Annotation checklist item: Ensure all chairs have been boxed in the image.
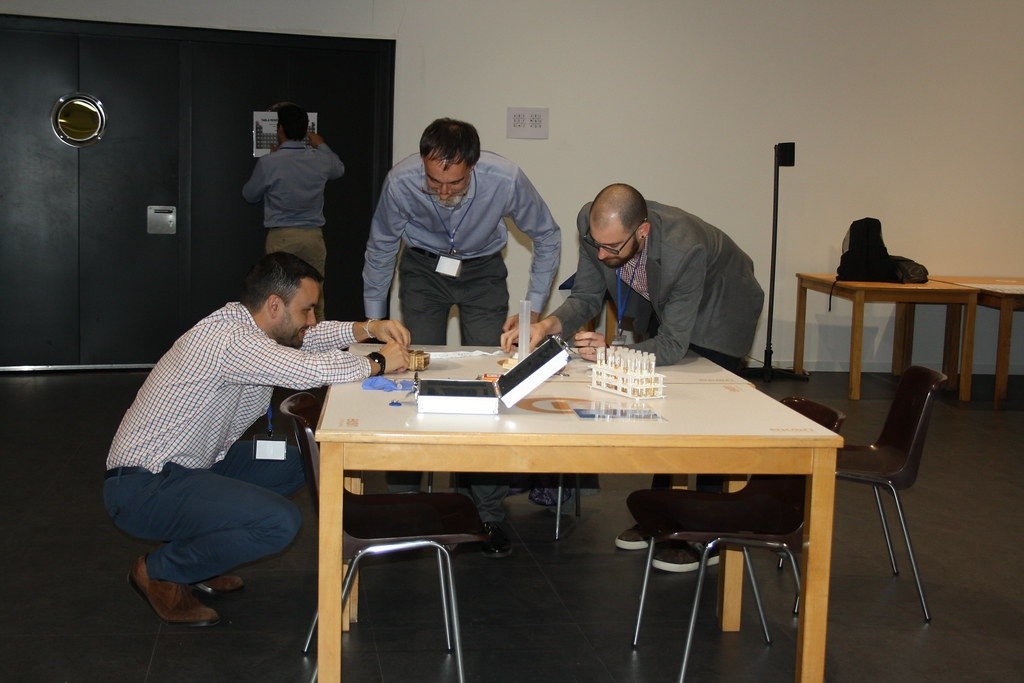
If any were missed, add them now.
[625,397,847,683]
[776,364,948,623]
[279,391,490,683]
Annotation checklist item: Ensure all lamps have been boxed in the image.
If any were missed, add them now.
[752,142,795,383]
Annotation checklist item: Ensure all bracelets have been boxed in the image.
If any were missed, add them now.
[364,319,378,338]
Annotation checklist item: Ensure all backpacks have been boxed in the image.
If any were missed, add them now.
[837,218,928,284]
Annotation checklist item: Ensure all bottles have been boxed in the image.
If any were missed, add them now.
[596,344,657,397]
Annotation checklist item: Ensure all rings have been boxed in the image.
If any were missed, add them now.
[592,347,596,354]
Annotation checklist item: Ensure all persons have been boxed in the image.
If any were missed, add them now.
[244,106,345,324]
[502,184,765,573]
[104,252,411,625]
[362,117,562,559]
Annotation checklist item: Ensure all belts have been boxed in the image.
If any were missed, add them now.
[103,467,150,479]
[409,246,483,263]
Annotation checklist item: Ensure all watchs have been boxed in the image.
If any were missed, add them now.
[367,352,385,376]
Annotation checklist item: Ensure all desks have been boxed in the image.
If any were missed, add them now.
[313,346,844,683]
[902,277,1024,411]
[793,272,982,401]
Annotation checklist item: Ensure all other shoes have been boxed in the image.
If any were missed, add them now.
[128,553,220,628]
[480,520,513,558]
[614,521,659,550]
[187,575,246,595]
[651,538,720,573]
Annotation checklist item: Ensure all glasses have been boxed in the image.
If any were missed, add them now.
[582,217,648,255]
[420,158,472,196]
